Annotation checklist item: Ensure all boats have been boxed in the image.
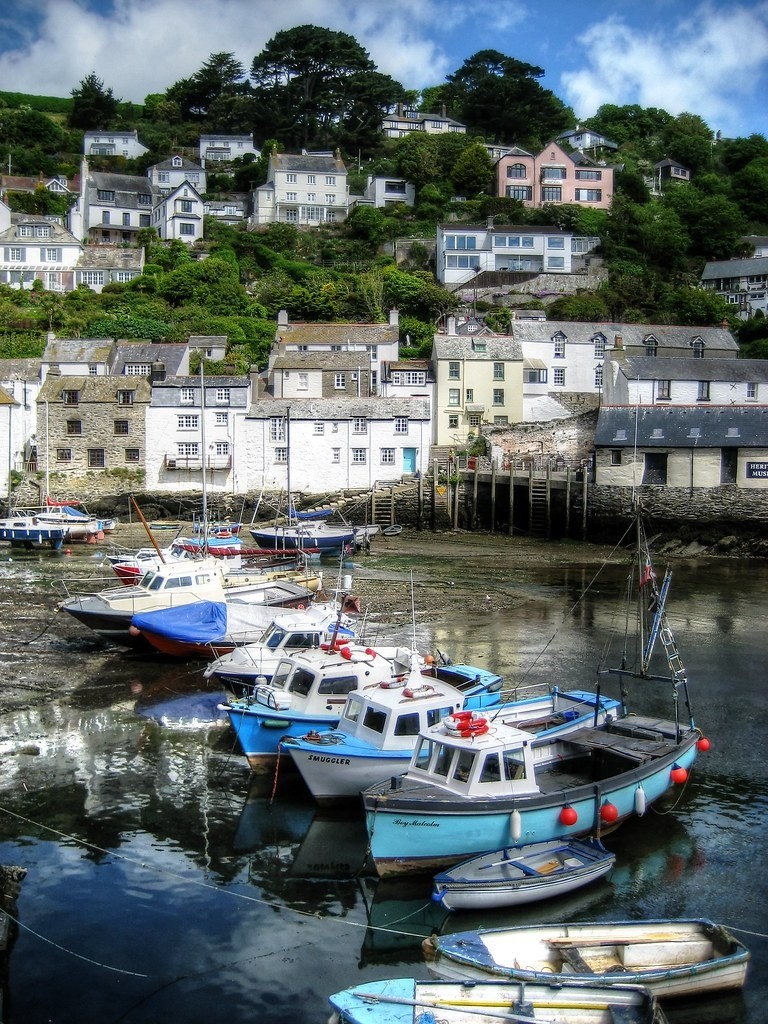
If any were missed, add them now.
[214,591,505,779]
[279,800,377,886]
[326,977,670,1024]
[357,799,700,969]
[438,876,617,943]
[430,833,616,926]
[228,769,317,856]
[359,373,708,885]
[420,916,754,1007]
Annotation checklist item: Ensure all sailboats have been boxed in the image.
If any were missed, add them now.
[280,566,621,812]
[0,395,460,735]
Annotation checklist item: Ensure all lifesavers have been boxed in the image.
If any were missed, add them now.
[403,684,434,698]
[379,677,409,689]
[216,531,230,538]
[342,645,377,662]
[321,638,355,650]
[445,710,489,737]
